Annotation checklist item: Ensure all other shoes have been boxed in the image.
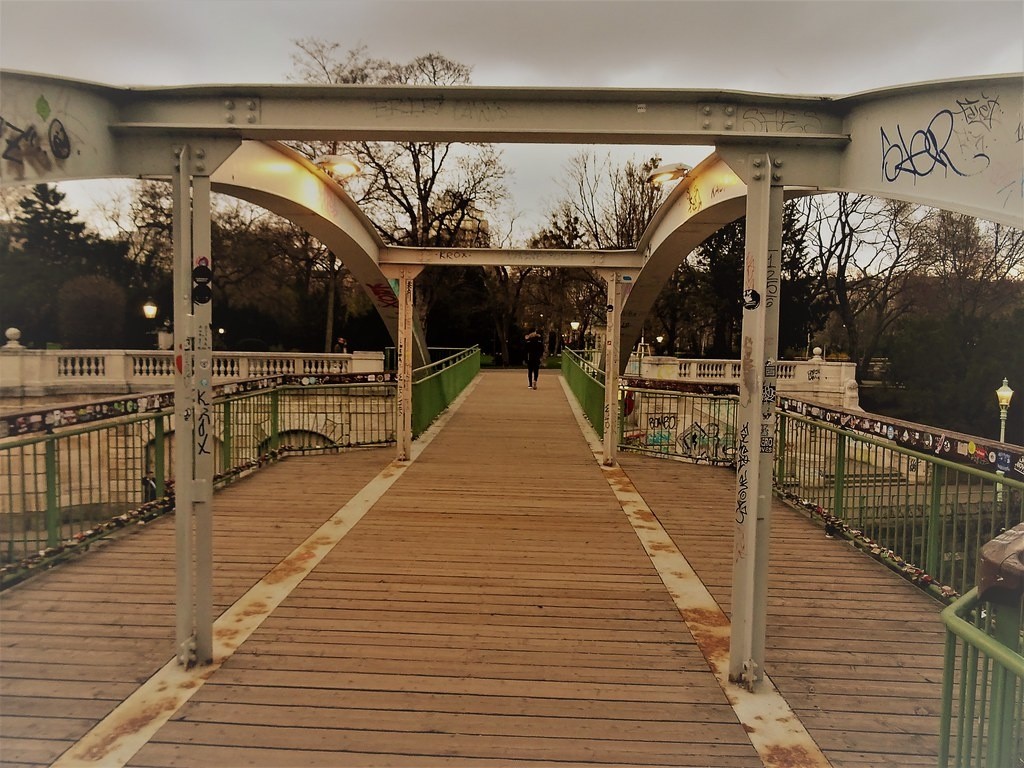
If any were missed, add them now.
[528,386,532,388]
[533,381,537,390]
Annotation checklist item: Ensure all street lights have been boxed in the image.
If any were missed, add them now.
[994,377,1013,513]
[570,317,580,352]
[312,155,361,352]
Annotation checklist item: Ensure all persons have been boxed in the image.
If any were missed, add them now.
[523,322,544,390]
[334,338,351,369]
